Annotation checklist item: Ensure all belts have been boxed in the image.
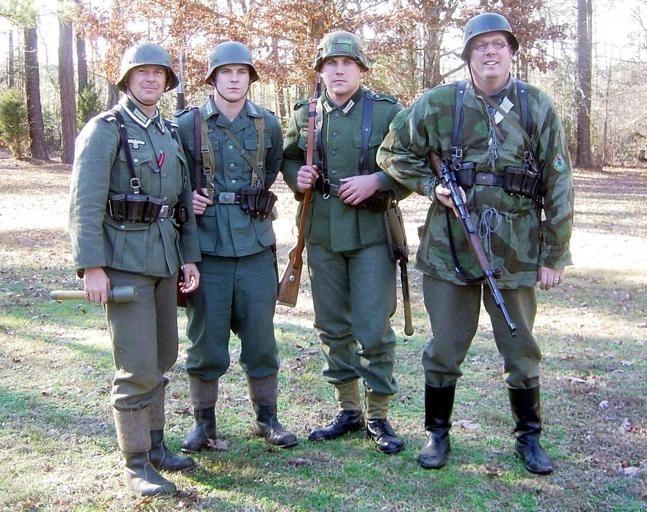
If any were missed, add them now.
[214,193,241,205]
[158,205,175,218]
[474,173,504,187]
[322,182,341,196]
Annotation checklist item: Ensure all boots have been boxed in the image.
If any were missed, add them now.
[418,382,455,469]
[508,384,554,475]
[367,389,404,454]
[308,379,365,440]
[113,394,196,496]
[246,374,299,448]
[181,375,218,453]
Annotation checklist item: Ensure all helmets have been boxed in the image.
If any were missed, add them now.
[461,13,519,60]
[204,42,259,85]
[115,42,179,92]
[313,32,369,72]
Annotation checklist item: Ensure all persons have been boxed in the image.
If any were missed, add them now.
[70,42,202,497]
[279,30,413,454]
[176,41,299,454]
[376,13,574,475]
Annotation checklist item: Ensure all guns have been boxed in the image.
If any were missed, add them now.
[429,151,518,337]
[274,71,321,307]
[173,55,187,307]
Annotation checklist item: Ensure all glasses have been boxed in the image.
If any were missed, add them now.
[473,39,505,51]
